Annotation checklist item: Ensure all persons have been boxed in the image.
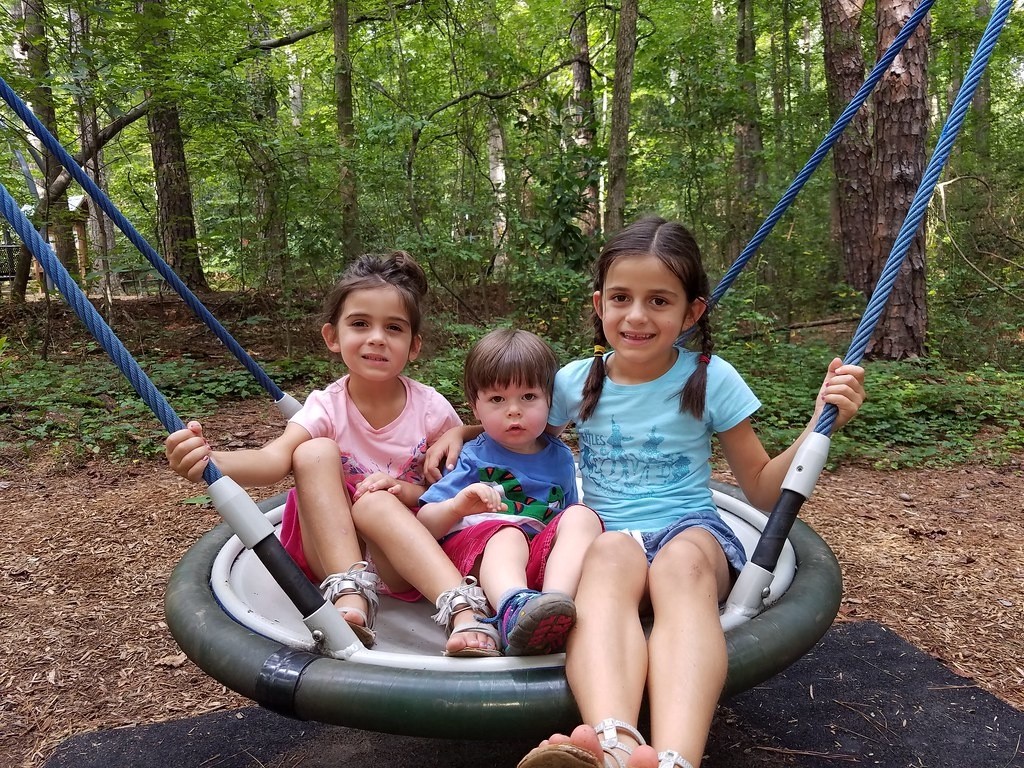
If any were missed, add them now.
[417,327,605,657]
[163,248,465,652]
[419,217,868,767]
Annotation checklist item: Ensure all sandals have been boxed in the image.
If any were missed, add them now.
[517,718,647,768]
[430,575,503,658]
[318,562,380,646]
[657,749,696,768]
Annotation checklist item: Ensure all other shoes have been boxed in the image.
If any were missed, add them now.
[473,588,577,657]
